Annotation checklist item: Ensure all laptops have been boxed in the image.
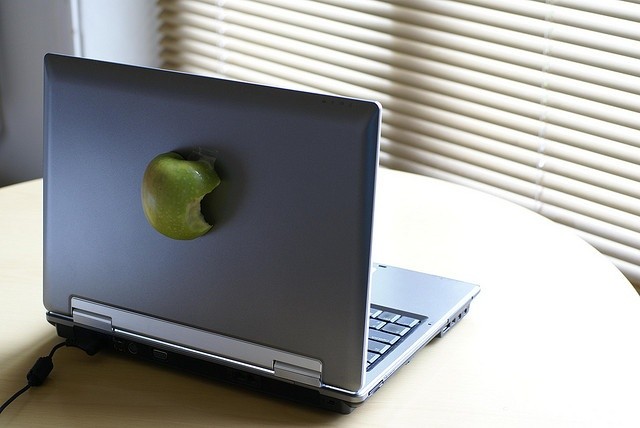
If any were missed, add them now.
[43,51,480,415]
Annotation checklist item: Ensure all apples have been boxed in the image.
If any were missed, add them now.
[141,152,221,240]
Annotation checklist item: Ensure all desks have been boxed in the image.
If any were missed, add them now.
[0,163,636,427]
